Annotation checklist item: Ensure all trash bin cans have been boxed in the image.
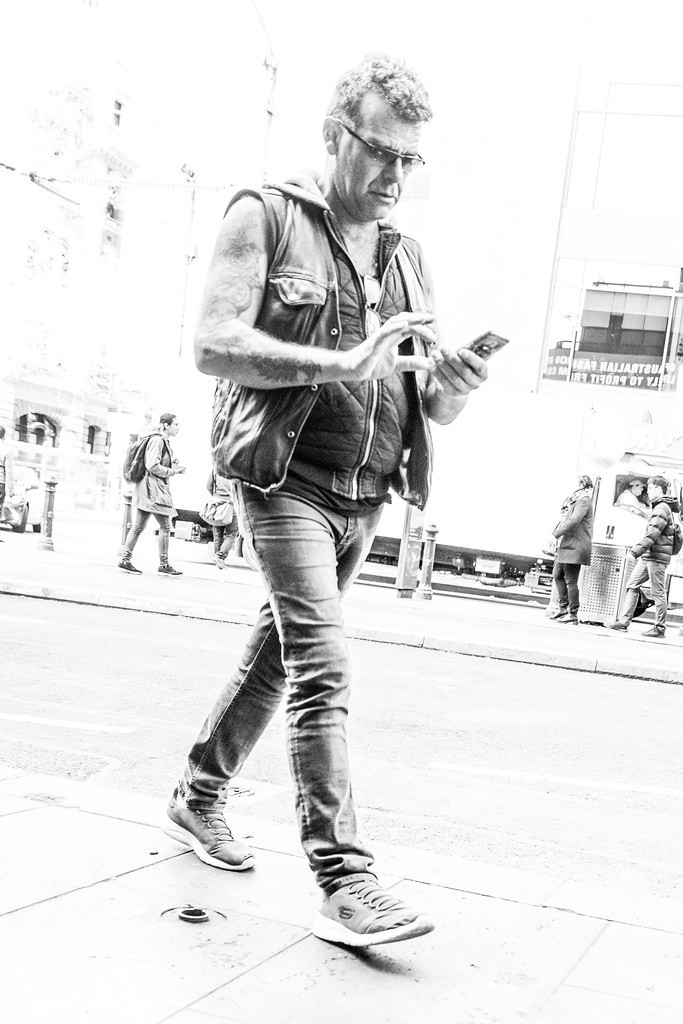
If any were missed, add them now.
[544,541,642,626]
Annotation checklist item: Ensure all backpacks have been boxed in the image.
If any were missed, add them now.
[123,437,150,484]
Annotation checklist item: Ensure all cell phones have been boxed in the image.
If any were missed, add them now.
[469,331,512,361]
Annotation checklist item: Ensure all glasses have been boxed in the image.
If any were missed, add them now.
[335,119,426,173]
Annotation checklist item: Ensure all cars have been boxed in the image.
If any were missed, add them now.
[1,461,50,534]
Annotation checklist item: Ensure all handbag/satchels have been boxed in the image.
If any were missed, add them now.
[672,524,683,555]
[541,520,561,558]
[199,497,233,526]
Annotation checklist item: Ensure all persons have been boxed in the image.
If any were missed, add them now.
[204,463,240,571]
[157,54,491,948]
[549,475,594,625]
[615,479,647,515]
[603,475,675,638]
[116,414,185,578]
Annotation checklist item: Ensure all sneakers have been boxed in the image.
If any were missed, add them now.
[313,880,435,946]
[161,786,256,871]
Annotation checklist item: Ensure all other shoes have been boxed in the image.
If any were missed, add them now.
[212,555,227,570]
[641,626,664,638]
[557,611,579,625]
[118,560,142,575]
[550,609,569,620]
[158,565,183,577]
[606,622,628,632]
